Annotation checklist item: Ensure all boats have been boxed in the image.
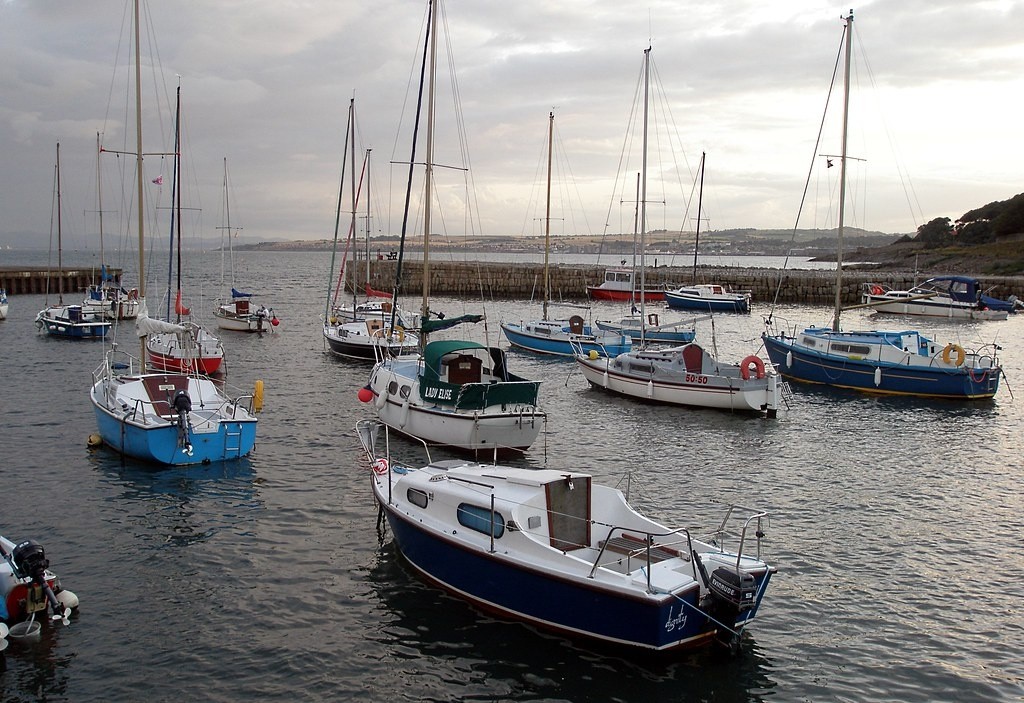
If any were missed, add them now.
[353,414,780,665]
[862,272,1024,322]
[0,292,11,320]
[1,536,79,643]
[585,267,679,300]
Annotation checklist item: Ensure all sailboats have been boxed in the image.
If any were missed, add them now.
[563,37,786,419]
[501,106,634,356]
[82,133,141,319]
[35,139,112,340]
[212,155,278,334]
[142,85,227,375]
[595,172,698,344]
[757,8,1006,406]
[92,0,259,468]
[323,90,423,360]
[665,148,752,315]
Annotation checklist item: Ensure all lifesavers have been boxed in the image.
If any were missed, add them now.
[388,326,404,342]
[253,380,263,413]
[870,286,883,295]
[943,344,966,366]
[741,355,764,379]
[128,288,138,299]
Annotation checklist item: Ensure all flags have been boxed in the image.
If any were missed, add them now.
[152,175,163,185]
